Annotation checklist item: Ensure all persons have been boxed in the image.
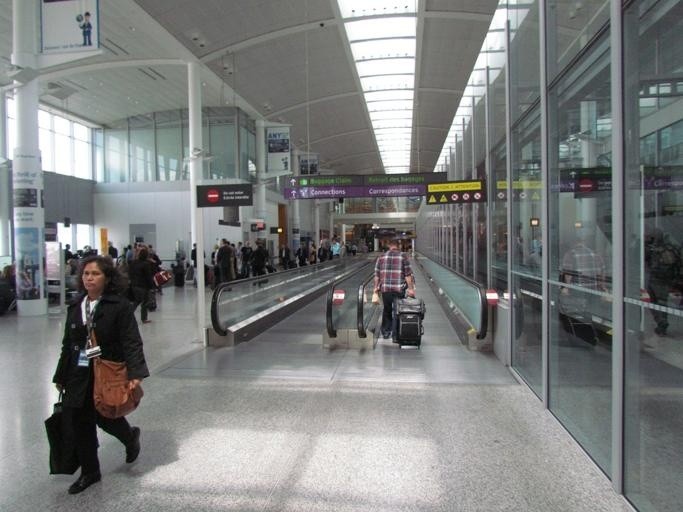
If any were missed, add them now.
[50,254,150,494]
[169,233,416,291]
[371,238,415,344]
[476,218,543,286]
[78,10,93,46]
[0,232,164,326]
[557,219,680,334]
[282,157,288,170]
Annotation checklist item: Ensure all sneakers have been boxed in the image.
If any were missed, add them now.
[142,320,152,324]
[384,330,399,343]
[654,322,668,334]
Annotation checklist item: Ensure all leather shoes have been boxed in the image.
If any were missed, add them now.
[126,427,141,463]
[69,473,100,494]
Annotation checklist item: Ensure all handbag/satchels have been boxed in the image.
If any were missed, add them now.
[153,270,172,287]
[44,388,100,476]
[93,356,144,419]
[396,298,425,319]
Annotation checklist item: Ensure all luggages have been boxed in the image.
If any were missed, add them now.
[397,313,422,350]
